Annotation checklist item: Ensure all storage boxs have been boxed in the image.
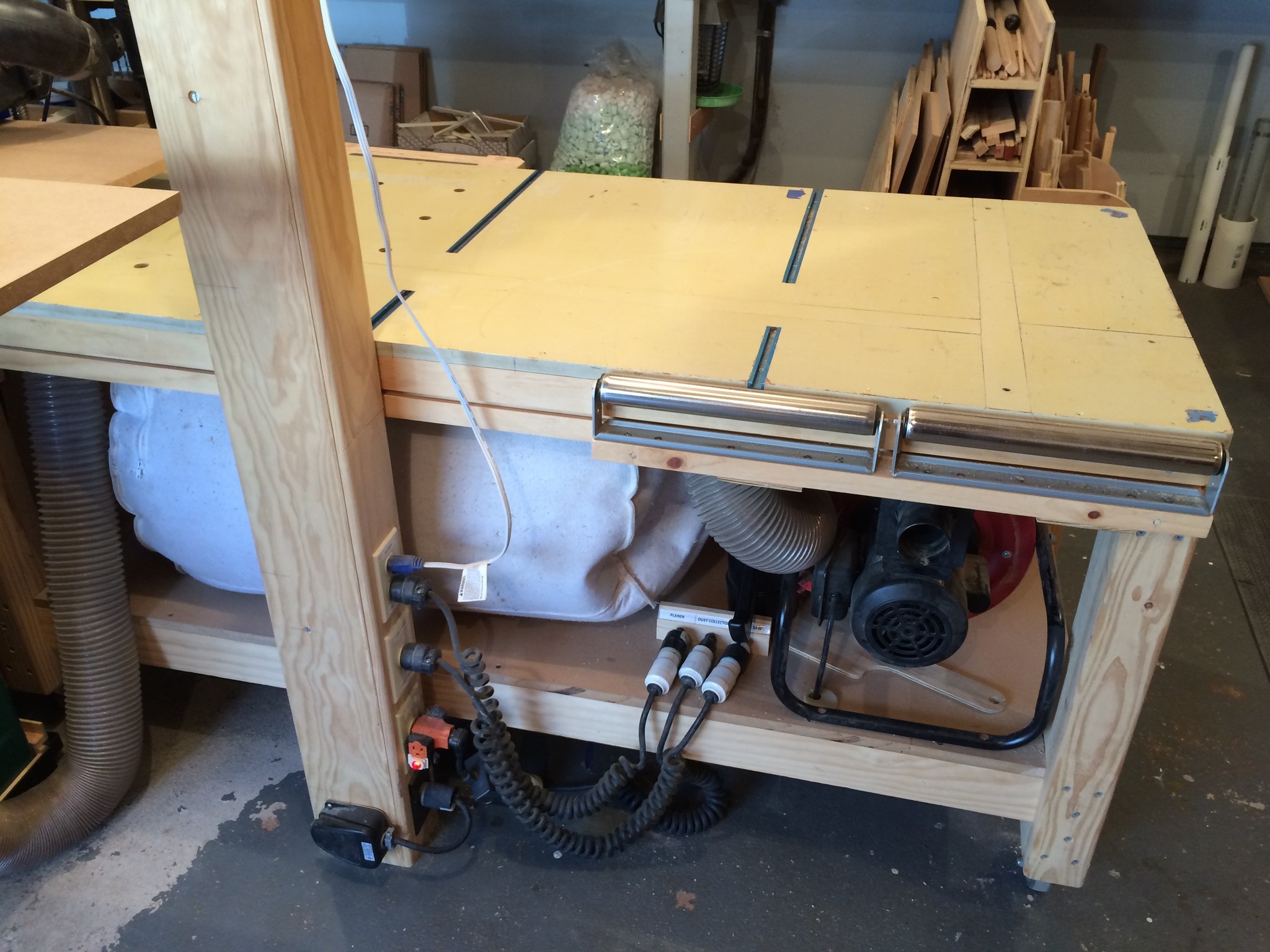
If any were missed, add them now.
[336,43,537,168]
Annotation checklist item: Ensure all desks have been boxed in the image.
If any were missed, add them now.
[0,179,182,313]
[0,122,167,184]
[0,122,1236,888]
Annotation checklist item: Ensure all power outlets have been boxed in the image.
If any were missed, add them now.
[372,527,402,623]
[396,681,420,778]
[384,606,417,704]
